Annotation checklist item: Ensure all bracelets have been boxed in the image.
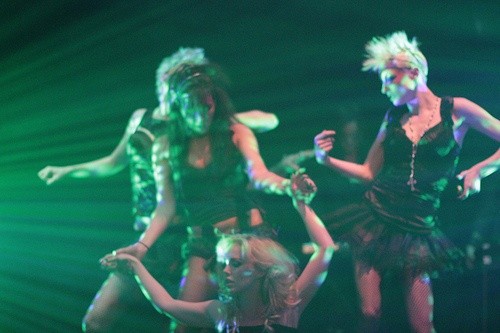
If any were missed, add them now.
[136,239,151,252]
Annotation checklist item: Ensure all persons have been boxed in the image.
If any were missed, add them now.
[38,48,336,333]
[313,31,500,333]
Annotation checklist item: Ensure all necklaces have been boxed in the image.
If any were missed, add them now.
[405,97,438,190]
